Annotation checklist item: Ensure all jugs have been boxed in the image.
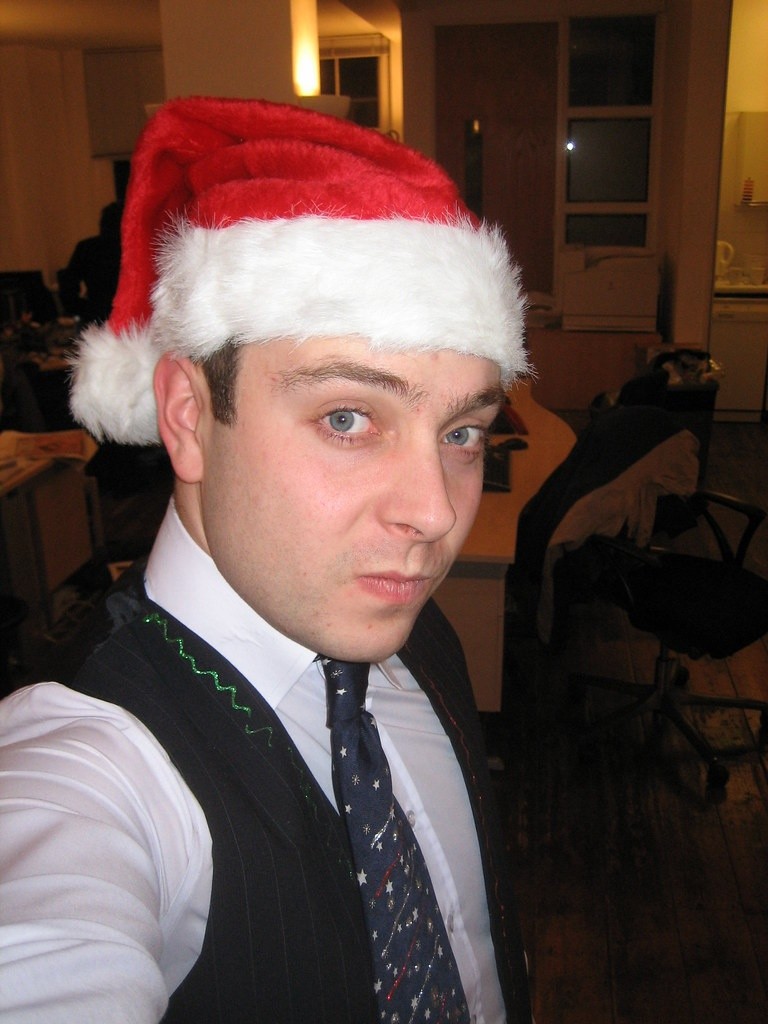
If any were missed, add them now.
[714,239,734,276]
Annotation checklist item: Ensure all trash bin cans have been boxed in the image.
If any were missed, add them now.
[431,372,578,710]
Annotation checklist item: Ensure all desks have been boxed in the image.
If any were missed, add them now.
[432,371,576,711]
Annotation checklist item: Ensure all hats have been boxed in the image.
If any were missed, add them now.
[68,97,529,445]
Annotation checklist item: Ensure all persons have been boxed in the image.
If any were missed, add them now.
[59,202,126,325]
[0,94,539,1024]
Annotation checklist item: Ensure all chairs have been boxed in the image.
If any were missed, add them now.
[562,409,768,789]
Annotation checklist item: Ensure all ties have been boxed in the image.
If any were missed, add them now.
[317,650,472,1024]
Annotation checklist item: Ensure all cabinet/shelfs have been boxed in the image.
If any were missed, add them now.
[554,13,668,333]
[709,297,768,421]
[0,429,106,638]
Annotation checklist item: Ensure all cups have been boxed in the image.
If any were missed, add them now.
[750,268,767,285]
[725,267,741,284]
